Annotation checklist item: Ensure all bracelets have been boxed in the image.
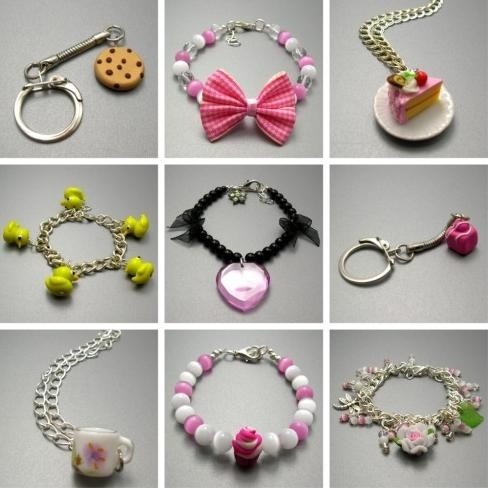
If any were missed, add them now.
[172,16,319,147]
[165,178,321,315]
[171,343,317,471]
[334,353,486,462]
[1,185,155,298]
